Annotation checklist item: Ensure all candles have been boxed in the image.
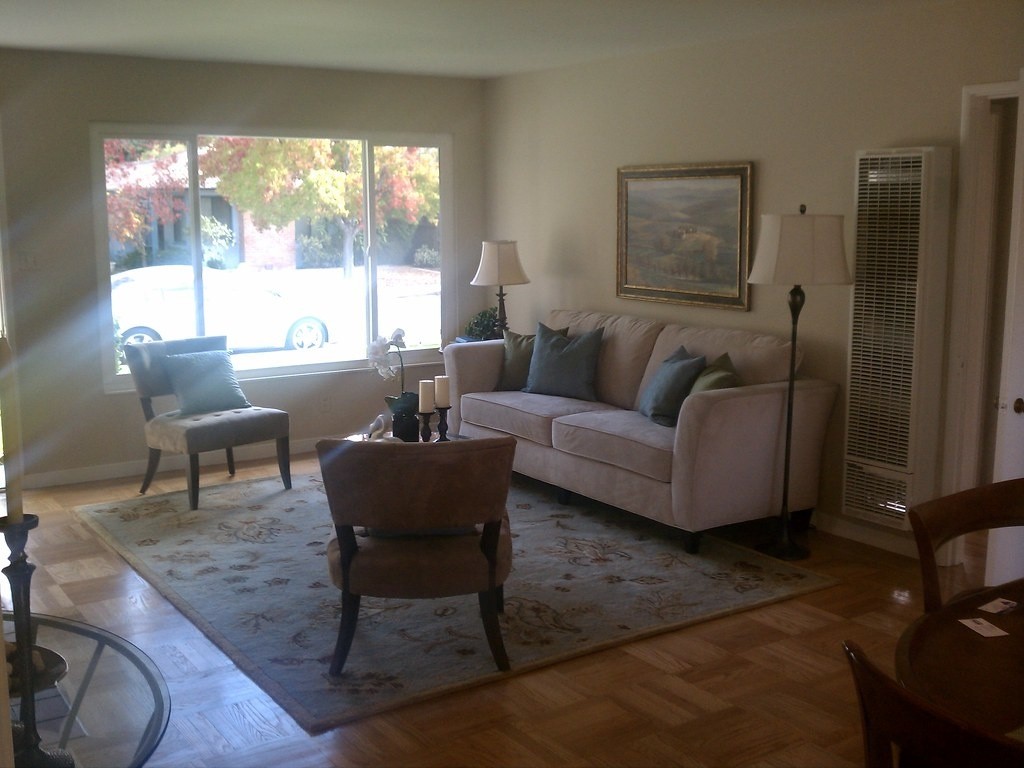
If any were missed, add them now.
[433,376,450,408]
[418,380,434,413]
[0,329,24,524]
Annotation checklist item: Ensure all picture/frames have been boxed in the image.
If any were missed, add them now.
[615,162,750,312]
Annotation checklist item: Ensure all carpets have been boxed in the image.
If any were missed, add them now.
[69,469,840,733]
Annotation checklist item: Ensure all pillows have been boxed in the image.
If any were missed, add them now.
[639,345,707,426]
[492,327,569,391]
[520,321,604,401]
[689,352,737,393]
[162,349,250,415]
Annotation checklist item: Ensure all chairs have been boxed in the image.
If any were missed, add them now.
[907,478,1024,612]
[121,336,291,510]
[315,436,516,673]
[841,639,1024,768]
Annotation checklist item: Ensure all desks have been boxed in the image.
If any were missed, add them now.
[894,590,1024,741]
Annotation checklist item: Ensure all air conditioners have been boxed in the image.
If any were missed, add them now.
[841,145,952,532]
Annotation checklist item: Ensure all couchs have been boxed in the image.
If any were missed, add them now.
[442,309,840,553]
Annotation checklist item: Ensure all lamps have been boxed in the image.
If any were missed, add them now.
[469,240,530,341]
[745,204,853,562]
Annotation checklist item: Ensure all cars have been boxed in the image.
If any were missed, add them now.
[111,264,337,365]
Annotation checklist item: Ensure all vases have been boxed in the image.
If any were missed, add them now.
[391,414,419,442]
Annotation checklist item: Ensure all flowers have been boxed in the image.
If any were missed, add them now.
[367,328,419,419]
